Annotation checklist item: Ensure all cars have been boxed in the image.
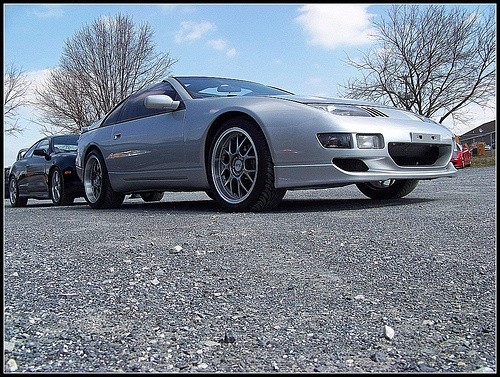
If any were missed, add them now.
[74,74,459,212]
[449,142,472,169]
[8,134,164,206]
